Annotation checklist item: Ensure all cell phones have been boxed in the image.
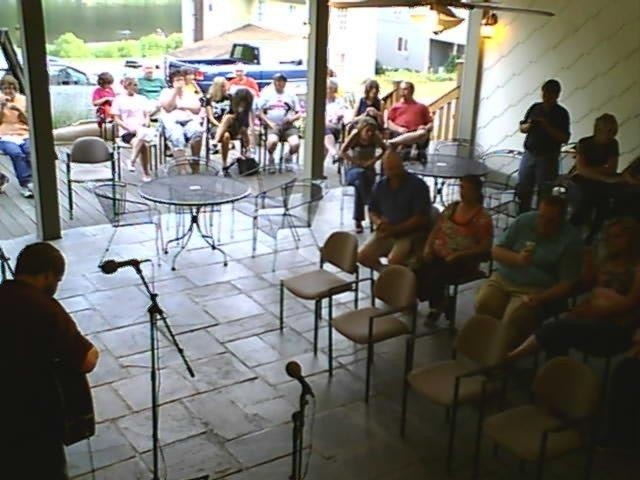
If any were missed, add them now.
[527,242,536,247]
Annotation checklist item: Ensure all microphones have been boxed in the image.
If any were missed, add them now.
[285,360,317,399]
[97,259,139,274]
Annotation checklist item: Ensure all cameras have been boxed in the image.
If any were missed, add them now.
[200,97,210,107]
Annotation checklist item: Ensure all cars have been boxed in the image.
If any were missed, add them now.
[45,55,116,126]
[120,55,165,90]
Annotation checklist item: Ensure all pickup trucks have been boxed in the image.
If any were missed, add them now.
[165,42,333,94]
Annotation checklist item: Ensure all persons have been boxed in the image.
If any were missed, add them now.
[518,77,570,210]
[508,218,638,364]
[93,64,300,175]
[336,115,386,234]
[324,68,433,177]
[0,243,99,480]
[1,73,36,198]
[569,112,637,204]
[473,195,598,334]
[357,150,431,272]
[416,173,494,325]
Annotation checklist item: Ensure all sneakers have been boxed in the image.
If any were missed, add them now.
[21,186,33,198]
[127,153,294,183]
[427,296,455,321]
[355,220,363,233]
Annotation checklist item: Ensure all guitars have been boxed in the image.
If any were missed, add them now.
[0,249,94,445]
